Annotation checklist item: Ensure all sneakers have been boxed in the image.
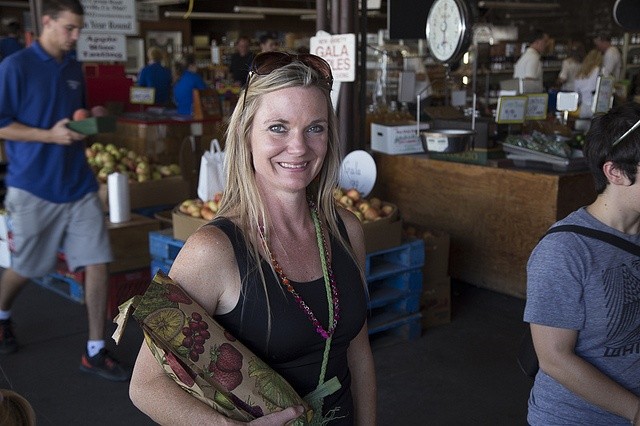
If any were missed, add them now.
[79,347,132,382]
[0,317,18,355]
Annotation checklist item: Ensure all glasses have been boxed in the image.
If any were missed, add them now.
[243,51,333,109]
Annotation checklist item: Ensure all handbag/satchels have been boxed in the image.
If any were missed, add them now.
[197,138,229,202]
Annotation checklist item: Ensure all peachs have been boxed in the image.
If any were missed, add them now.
[72,109,89,121]
[91,106,109,118]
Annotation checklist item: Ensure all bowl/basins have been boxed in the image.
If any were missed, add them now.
[419,128,474,153]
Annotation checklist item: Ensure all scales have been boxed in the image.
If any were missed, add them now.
[420,0,478,154]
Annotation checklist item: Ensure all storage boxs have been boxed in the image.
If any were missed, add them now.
[356,204,404,252]
[170,204,210,239]
[420,280,450,327]
[86,143,189,209]
[409,225,448,282]
[370,119,430,154]
[106,214,162,275]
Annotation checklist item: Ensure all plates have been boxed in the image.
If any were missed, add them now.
[339,151,376,198]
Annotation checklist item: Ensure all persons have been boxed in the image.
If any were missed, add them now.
[572,47,609,120]
[128,50,379,426]
[137,45,172,104]
[171,57,209,115]
[0,21,22,59]
[510,29,549,92]
[258,33,279,52]
[592,31,622,81]
[523,100,639,426]
[0,1,132,383]
[227,37,255,83]
[554,39,585,90]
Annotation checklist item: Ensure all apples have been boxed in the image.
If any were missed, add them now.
[406,225,432,242]
[333,189,392,223]
[177,194,225,219]
[86,143,180,180]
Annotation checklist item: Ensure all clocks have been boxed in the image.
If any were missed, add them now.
[425,0,472,69]
